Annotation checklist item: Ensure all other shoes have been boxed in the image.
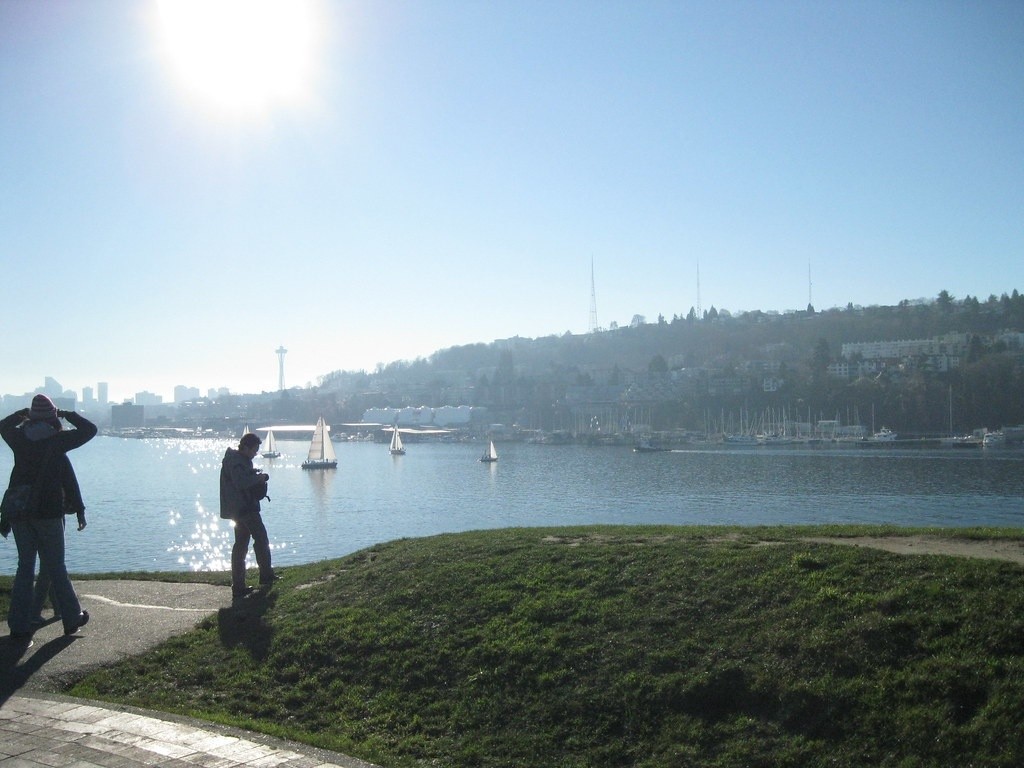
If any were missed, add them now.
[65,611,89,634]
[233,585,253,597]
[54,612,62,618]
[260,576,283,584]
[10,630,24,637]
[32,615,45,624]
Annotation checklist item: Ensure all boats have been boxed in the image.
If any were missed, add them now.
[917,383,1009,447]
[631,433,672,453]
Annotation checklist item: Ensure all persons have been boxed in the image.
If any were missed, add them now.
[220,433,282,597]
[0,394,98,638]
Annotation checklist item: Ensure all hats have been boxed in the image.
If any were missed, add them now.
[30,394,57,420]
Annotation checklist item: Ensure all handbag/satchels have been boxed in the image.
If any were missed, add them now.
[252,481,267,500]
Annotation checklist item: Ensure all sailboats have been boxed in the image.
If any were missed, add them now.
[259,429,281,458]
[301,415,339,468]
[698,401,898,445]
[389,423,408,456]
[478,441,499,462]
